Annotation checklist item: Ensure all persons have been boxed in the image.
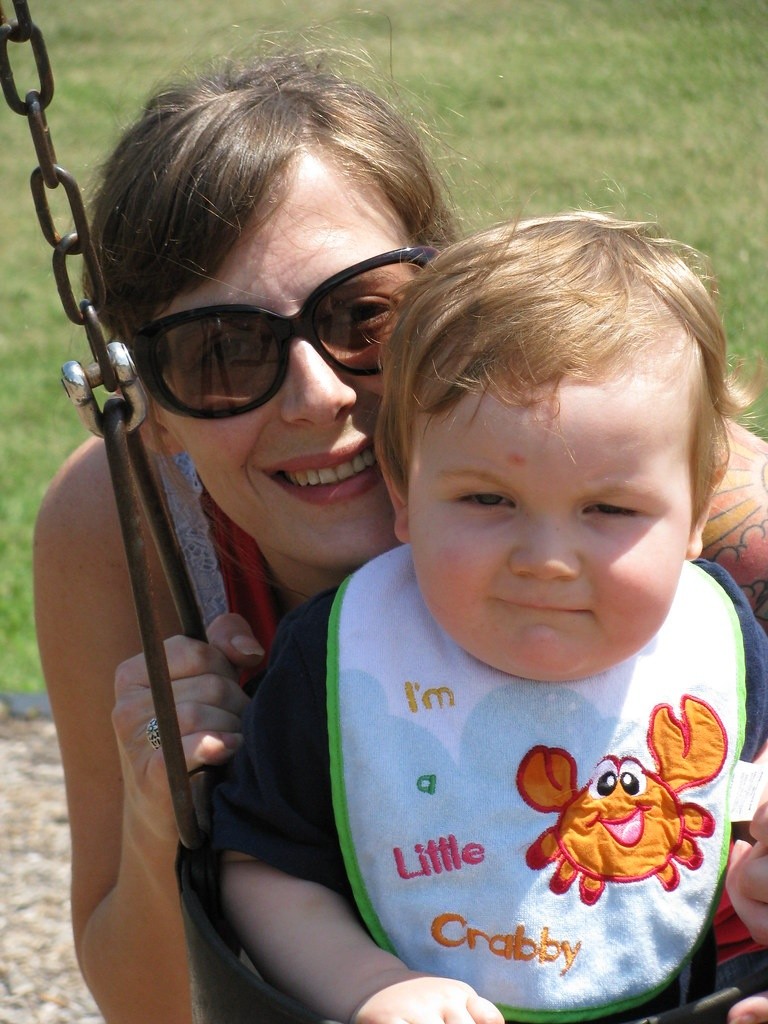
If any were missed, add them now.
[205,210,767,1024]
[31,68,768,1024]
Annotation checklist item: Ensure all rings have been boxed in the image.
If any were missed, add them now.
[146,719,161,750]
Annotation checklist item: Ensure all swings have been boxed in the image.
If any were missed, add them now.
[0,0,768,1024]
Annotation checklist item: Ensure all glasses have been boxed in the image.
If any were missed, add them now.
[129,246,441,420]
